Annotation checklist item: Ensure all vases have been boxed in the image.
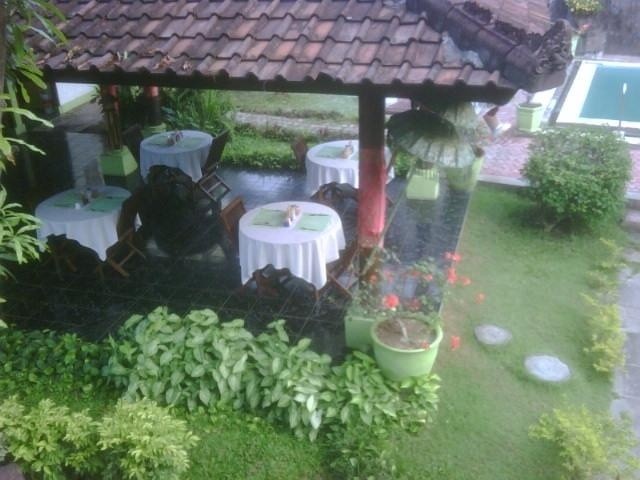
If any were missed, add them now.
[345,306,395,352]
[516,100,543,135]
[445,142,486,193]
[371,313,443,382]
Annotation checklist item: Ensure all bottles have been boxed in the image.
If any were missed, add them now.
[168,129,184,144]
[75,188,92,209]
[283,202,301,228]
[342,141,355,158]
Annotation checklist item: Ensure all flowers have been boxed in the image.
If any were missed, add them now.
[348,223,399,317]
[361,247,487,353]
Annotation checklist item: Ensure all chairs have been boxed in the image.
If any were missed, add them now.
[290,136,327,202]
[350,143,400,210]
[310,234,361,302]
[221,198,281,299]
[19,121,234,283]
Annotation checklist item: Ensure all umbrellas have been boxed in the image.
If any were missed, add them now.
[385,109,475,170]
[412,97,479,129]
